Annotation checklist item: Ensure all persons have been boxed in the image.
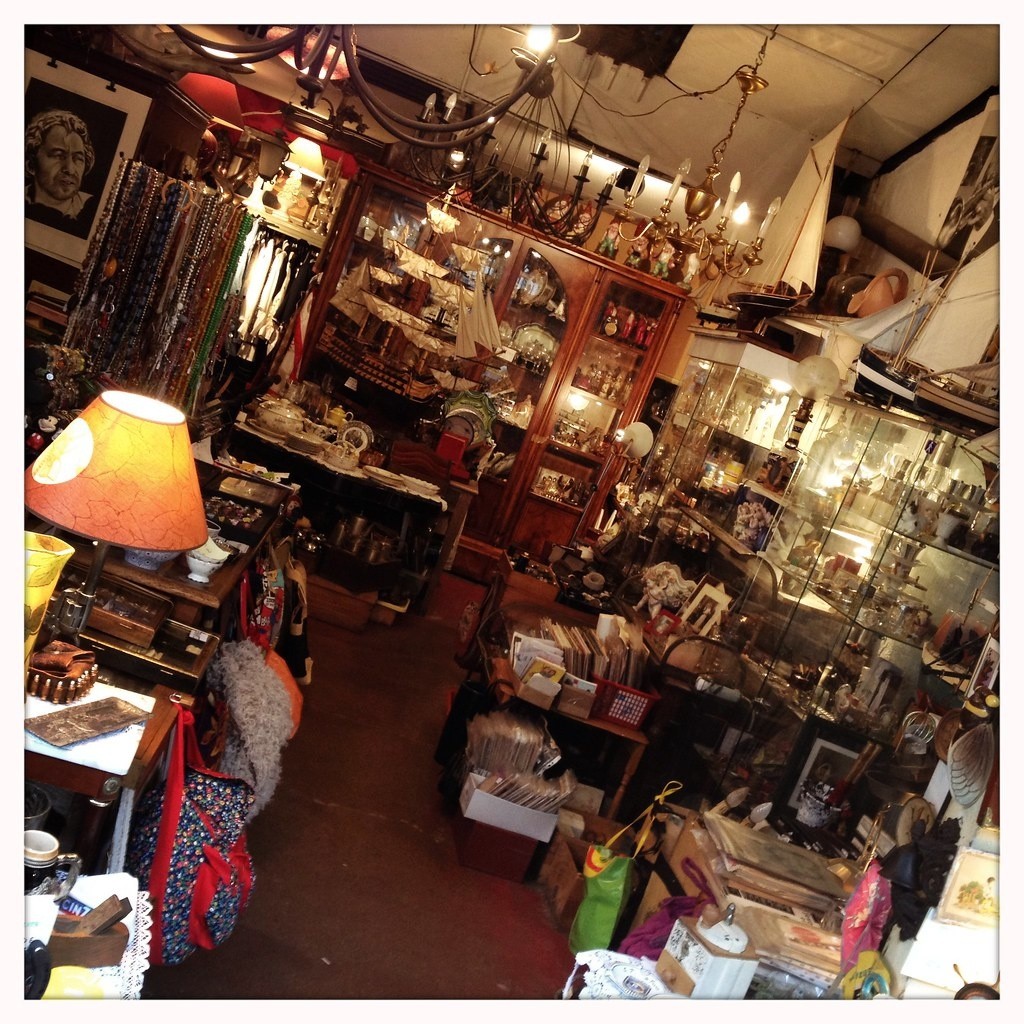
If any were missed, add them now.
[653,244,675,281]
[597,222,620,258]
[565,212,591,238]
[548,201,568,223]
[625,235,649,269]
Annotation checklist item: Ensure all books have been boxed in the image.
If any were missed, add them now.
[538,616,651,695]
[508,630,569,697]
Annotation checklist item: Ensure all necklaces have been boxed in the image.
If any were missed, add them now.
[61,153,253,414]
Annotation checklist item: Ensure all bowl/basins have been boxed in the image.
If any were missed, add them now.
[254,398,304,435]
[124,546,181,571]
[185,553,223,584]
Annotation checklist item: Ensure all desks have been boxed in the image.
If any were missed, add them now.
[229,412,446,636]
[470,620,649,821]
[23,675,196,875]
[52,471,300,692]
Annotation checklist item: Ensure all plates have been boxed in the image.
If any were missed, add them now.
[511,322,559,358]
[362,465,439,497]
[246,418,288,440]
[335,420,375,456]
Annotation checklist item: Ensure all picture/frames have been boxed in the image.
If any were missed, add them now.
[25,25,154,274]
[675,571,739,639]
[965,634,1000,698]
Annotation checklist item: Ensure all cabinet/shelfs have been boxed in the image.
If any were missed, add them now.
[781,486,1000,825]
[729,389,940,821]
[295,163,687,576]
[611,353,762,550]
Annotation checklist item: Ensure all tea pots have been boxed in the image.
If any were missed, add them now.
[322,403,355,430]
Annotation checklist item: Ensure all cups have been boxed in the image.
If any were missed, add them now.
[282,378,308,405]
[366,548,378,561]
[24,829,82,896]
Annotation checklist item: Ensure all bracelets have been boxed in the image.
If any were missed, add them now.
[47,380,80,410]
[30,342,93,379]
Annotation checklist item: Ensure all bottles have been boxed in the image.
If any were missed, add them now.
[341,516,366,557]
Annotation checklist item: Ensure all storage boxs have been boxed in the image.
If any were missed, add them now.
[541,840,639,931]
[558,652,595,723]
[508,636,556,711]
[459,824,538,883]
[455,773,558,845]
[596,665,659,728]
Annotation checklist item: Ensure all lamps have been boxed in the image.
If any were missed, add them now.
[180,26,862,314]
[765,357,840,487]
[26,391,209,703]
